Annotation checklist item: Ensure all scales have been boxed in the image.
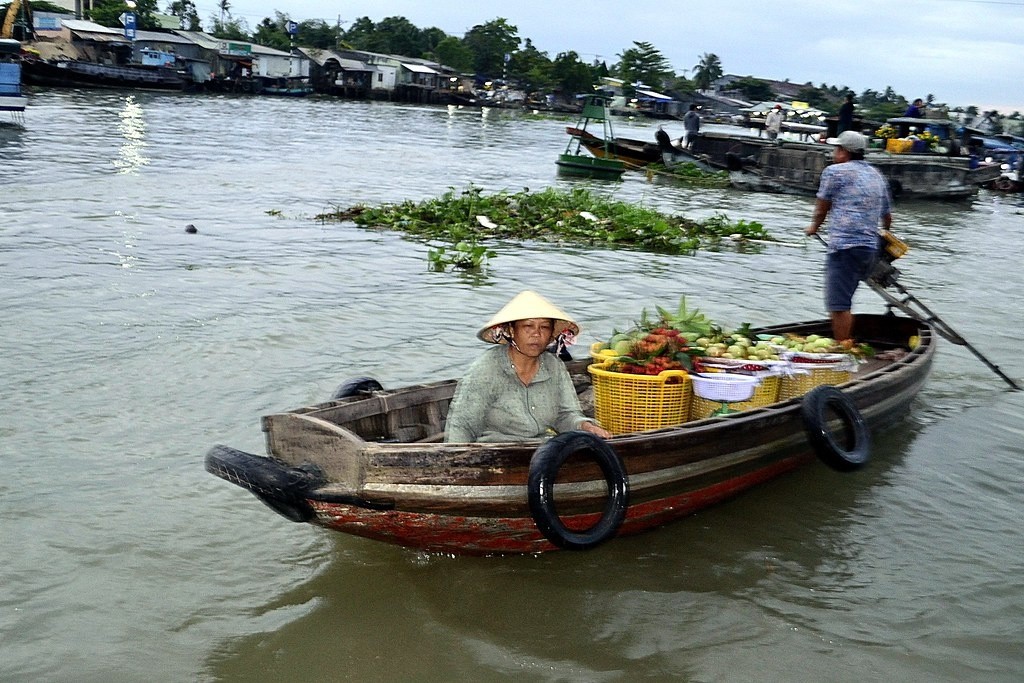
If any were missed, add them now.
[701,394,752,419]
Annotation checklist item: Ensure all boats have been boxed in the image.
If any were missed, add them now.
[656,124,798,193]
[447,91,526,116]
[15,54,187,93]
[563,124,677,169]
[260,79,313,95]
[706,110,1024,206]
[0,38,28,111]
[202,309,939,558]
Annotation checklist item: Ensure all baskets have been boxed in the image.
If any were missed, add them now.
[589,340,613,363]
[687,371,761,402]
[689,359,852,420]
[587,356,692,436]
[878,229,909,263]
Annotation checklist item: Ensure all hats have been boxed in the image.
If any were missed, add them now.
[476,290,582,346]
[774,104,782,110]
[689,104,699,110]
[825,131,867,154]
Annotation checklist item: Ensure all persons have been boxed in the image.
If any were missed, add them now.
[765,105,782,139]
[897,99,923,138]
[444,291,613,443]
[683,104,700,141]
[805,130,891,340]
[838,94,856,136]
